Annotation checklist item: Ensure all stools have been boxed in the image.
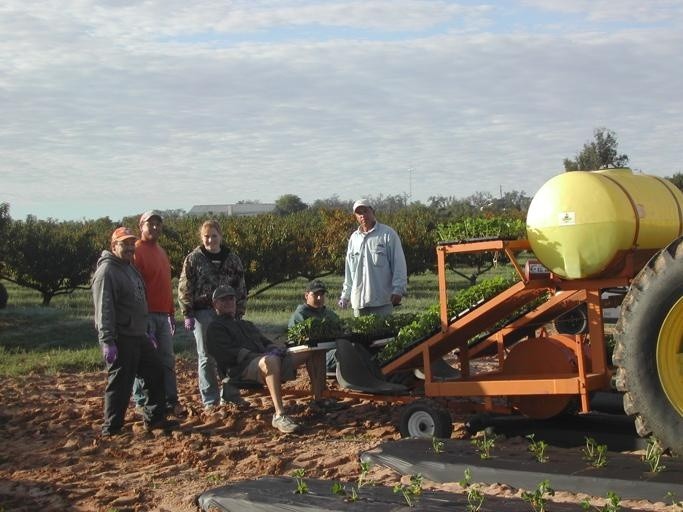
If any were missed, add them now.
[217,379,259,402]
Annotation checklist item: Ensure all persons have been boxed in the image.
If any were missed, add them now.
[92,227,180,438]
[287,280,340,372]
[205,285,346,434]
[129,210,186,416]
[338,199,407,329]
[177,220,250,413]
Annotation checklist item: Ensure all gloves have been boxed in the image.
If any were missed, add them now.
[168,312,176,336]
[147,331,160,353]
[103,342,118,364]
[185,318,194,330]
[264,348,283,357]
[338,299,347,309]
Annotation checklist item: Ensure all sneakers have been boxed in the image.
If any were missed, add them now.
[103,423,128,437]
[316,400,345,410]
[167,403,186,416]
[272,412,300,433]
[204,401,220,414]
[326,371,336,377]
[147,419,179,430]
[135,405,144,419]
[225,399,250,408]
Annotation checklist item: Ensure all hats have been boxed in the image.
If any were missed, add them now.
[353,199,369,213]
[212,285,237,300]
[306,280,328,293]
[111,226,139,243]
[139,210,164,224]
